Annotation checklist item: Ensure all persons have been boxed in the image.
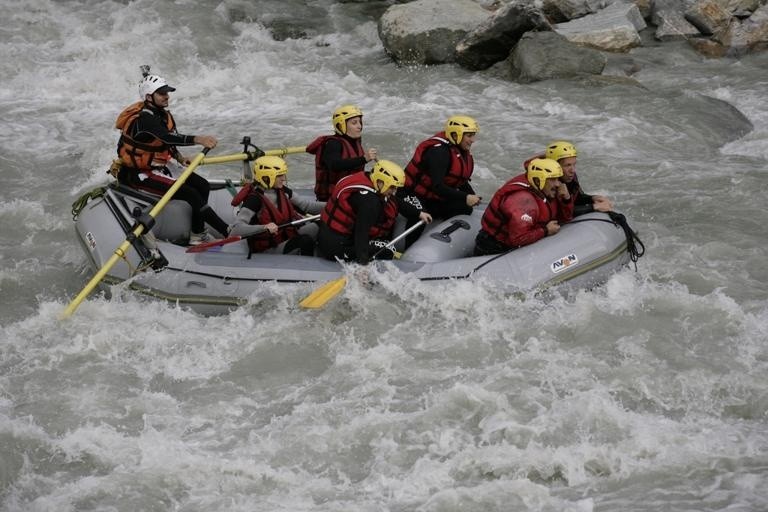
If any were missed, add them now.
[471,156,575,258]
[394,115,482,244]
[318,158,434,266]
[229,152,329,259]
[524,140,614,216]
[307,103,378,202]
[113,74,233,248]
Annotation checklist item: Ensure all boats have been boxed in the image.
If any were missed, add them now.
[72,180,644,318]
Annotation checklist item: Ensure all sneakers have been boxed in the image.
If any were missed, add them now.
[190,231,220,245]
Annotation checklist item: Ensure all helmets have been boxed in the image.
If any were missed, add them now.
[528,159,563,190]
[445,116,478,145]
[370,160,405,194]
[545,142,576,161]
[252,156,288,189]
[139,75,176,101]
[332,106,363,136]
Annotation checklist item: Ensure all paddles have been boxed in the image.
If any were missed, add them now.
[185,214,322,253]
[299,217,431,309]
